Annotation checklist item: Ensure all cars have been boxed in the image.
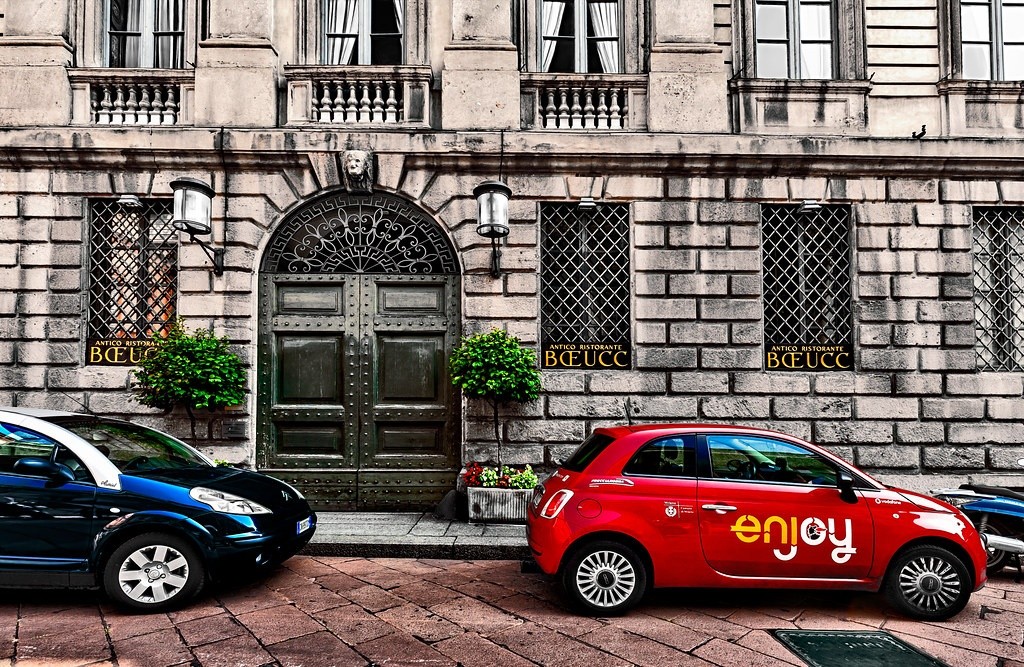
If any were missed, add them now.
[520,424,987,623]
[0,405,317,610]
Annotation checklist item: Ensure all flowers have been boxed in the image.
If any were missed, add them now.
[461,461,537,489]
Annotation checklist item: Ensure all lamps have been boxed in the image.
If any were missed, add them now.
[168,175,223,278]
[472,179,513,280]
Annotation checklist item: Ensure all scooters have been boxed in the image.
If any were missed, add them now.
[927,457,1024,576]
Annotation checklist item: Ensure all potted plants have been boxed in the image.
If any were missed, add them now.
[448,330,538,524]
